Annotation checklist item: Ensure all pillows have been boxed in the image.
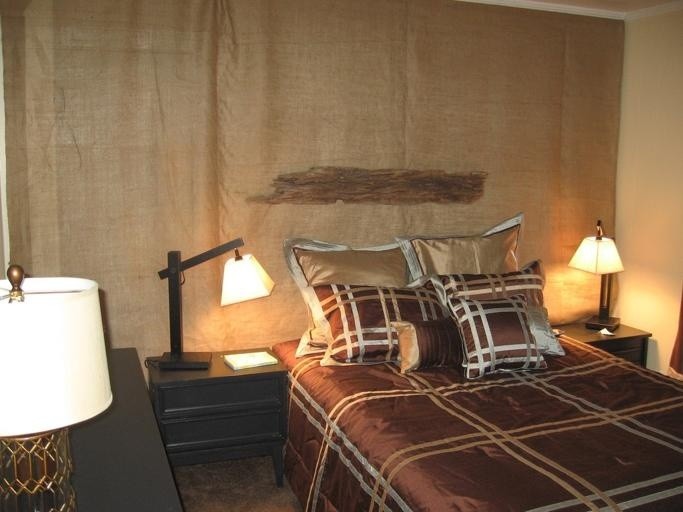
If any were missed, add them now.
[282,236,413,359]
[306,274,449,367]
[447,293,546,379]
[439,259,543,309]
[391,319,464,373]
[393,212,524,281]
[526,306,565,356]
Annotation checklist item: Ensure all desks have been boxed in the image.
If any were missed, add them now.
[69,347,183,512]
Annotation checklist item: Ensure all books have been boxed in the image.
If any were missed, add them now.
[224,351,278,370]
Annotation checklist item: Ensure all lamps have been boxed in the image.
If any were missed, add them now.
[158,237,275,369]
[567,219,624,332]
[0,263,113,512]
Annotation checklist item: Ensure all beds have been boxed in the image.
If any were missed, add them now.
[272,330,683,512]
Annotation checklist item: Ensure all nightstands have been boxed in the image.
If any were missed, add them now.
[147,347,289,487]
[549,323,653,369]
